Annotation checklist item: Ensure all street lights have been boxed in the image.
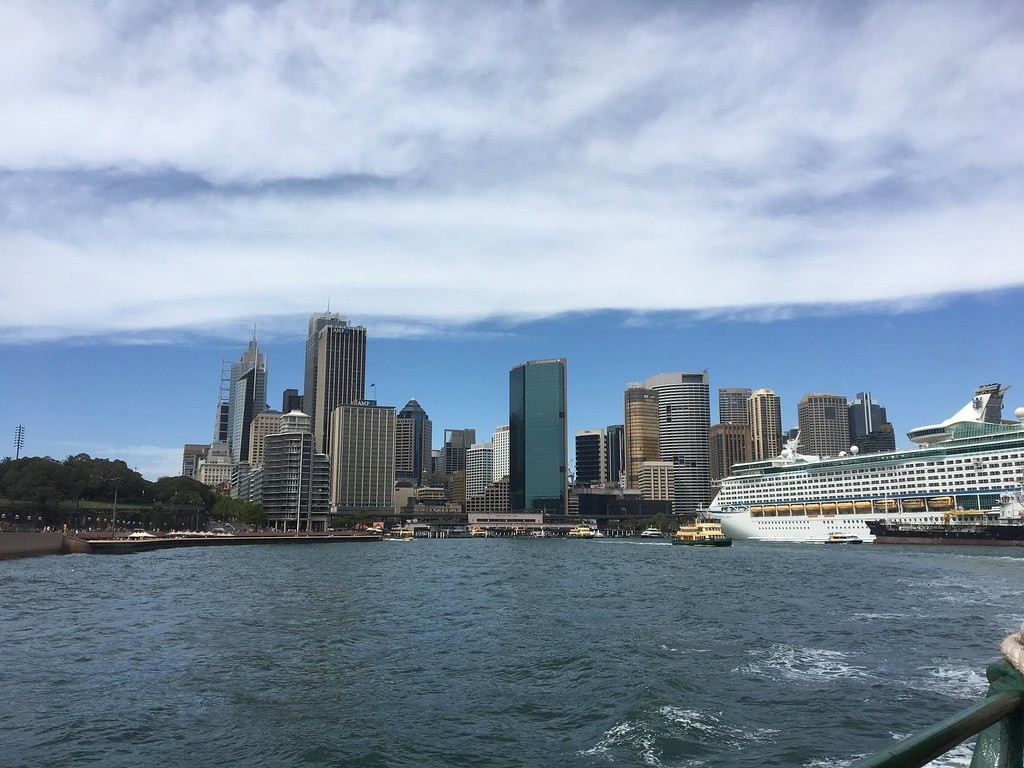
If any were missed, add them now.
[1,514,206,533]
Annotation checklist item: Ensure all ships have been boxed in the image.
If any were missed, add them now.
[705,385,1024,545]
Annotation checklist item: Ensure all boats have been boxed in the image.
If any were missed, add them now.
[674,514,734,547]
[823,531,864,545]
[403,536,415,541]
[565,523,596,539]
[390,525,412,539]
[467,523,486,538]
[640,524,665,539]
[865,510,1023,547]
[530,530,546,540]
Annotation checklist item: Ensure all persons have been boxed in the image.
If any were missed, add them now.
[41,524,298,542]
